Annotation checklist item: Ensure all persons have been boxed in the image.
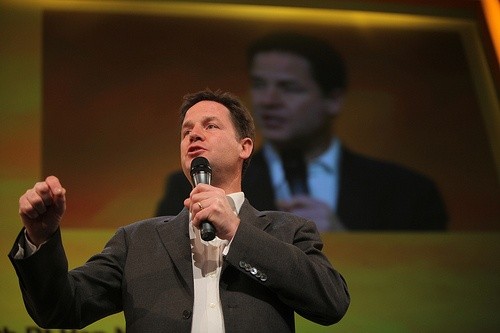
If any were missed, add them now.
[153,32,450,232]
[8,87,350,333]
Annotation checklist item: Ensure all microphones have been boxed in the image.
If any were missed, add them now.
[278,146,311,198]
[189,156,217,242]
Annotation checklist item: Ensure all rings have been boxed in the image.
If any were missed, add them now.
[196,201,203,211]
